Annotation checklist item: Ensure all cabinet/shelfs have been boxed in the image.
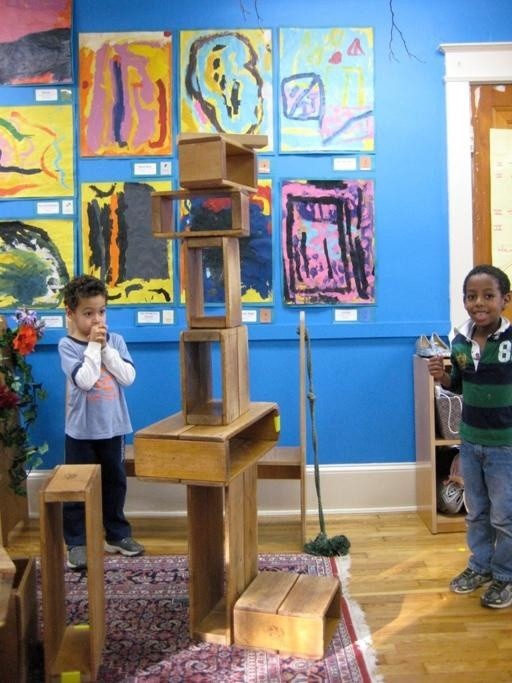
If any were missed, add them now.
[412,352,472,535]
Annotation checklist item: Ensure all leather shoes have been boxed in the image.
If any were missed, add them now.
[415,332,451,358]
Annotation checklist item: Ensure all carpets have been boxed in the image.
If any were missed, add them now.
[13,548,380,683]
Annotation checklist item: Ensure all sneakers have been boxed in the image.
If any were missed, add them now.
[66,545,86,569]
[481,578,512,608]
[105,537,144,556]
[449,568,492,593]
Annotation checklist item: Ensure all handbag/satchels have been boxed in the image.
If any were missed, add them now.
[434,385,464,440]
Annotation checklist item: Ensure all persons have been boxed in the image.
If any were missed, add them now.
[58,274,144,572]
[426,264,510,609]
